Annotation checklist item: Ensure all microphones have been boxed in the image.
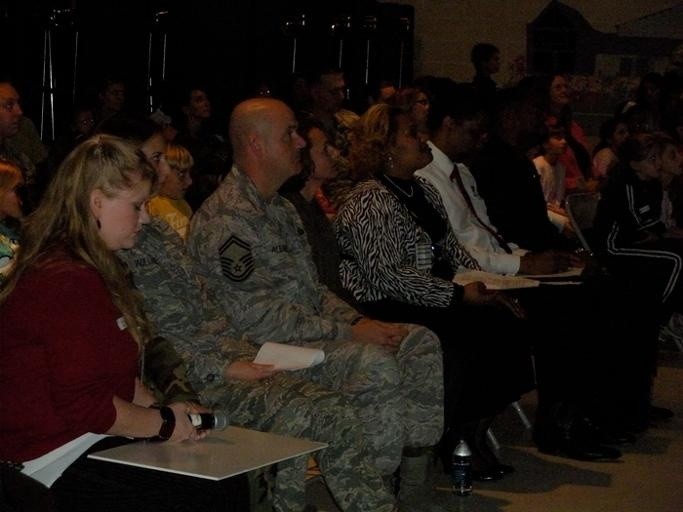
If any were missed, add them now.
[188,410,230,432]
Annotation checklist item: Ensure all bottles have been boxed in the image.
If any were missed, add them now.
[453,437,473,496]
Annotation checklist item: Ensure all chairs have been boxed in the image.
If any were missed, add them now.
[564,192,603,255]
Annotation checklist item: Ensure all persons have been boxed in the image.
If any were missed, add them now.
[0,43,683,512]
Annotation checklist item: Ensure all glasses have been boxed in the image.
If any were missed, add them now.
[413,98,429,105]
[404,127,420,139]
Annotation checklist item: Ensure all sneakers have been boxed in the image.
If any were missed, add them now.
[663,326,683,337]
[535,416,623,460]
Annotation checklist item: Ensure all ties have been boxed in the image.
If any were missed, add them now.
[449,163,513,255]
[658,333,682,354]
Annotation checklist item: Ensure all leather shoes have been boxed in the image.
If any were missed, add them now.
[649,406,673,420]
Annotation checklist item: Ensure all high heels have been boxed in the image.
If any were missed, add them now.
[433,440,454,475]
